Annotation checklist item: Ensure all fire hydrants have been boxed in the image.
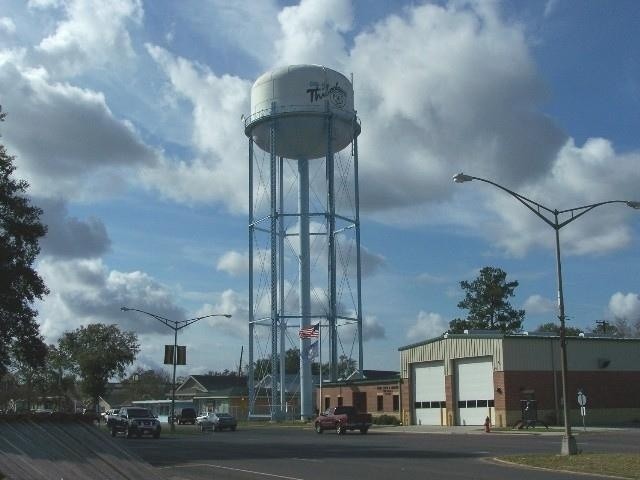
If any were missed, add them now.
[483,416,492,433]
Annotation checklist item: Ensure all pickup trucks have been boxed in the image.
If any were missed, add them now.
[107,407,161,439]
[314,405,372,436]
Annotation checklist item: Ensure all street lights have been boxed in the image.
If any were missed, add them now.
[452,172,640,460]
[120,306,232,433]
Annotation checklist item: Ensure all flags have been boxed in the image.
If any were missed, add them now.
[299,323,319,338]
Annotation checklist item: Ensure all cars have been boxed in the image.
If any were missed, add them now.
[104,409,120,422]
[168,407,236,432]
